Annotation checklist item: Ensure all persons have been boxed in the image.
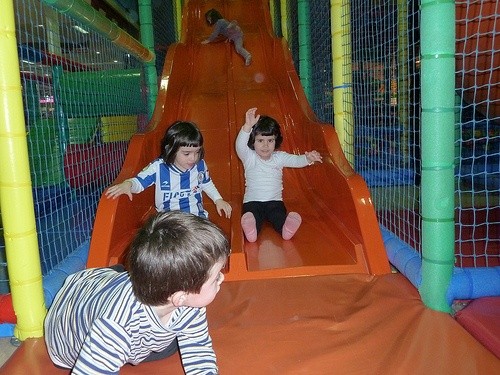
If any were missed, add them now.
[105,119,233,223]
[44,210,231,375]
[200,9,253,66]
[235,107,324,243]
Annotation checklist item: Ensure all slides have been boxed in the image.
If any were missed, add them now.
[84,0,391,281]
